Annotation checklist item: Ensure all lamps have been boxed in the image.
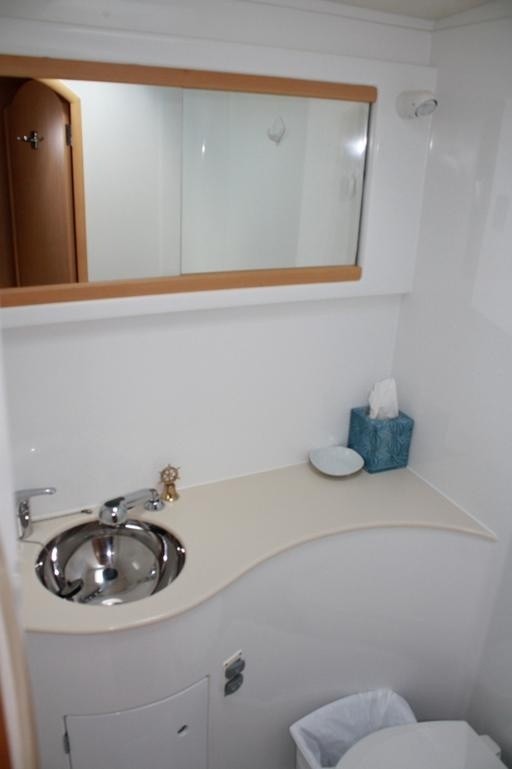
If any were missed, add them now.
[396,91,439,120]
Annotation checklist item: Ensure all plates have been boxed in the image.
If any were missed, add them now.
[308,444,367,479]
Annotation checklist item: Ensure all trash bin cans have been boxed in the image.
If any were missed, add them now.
[288,688,418,769]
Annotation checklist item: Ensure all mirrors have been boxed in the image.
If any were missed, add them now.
[0,53,377,308]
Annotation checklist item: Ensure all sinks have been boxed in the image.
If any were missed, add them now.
[40,518,186,608]
[97,488,161,523]
